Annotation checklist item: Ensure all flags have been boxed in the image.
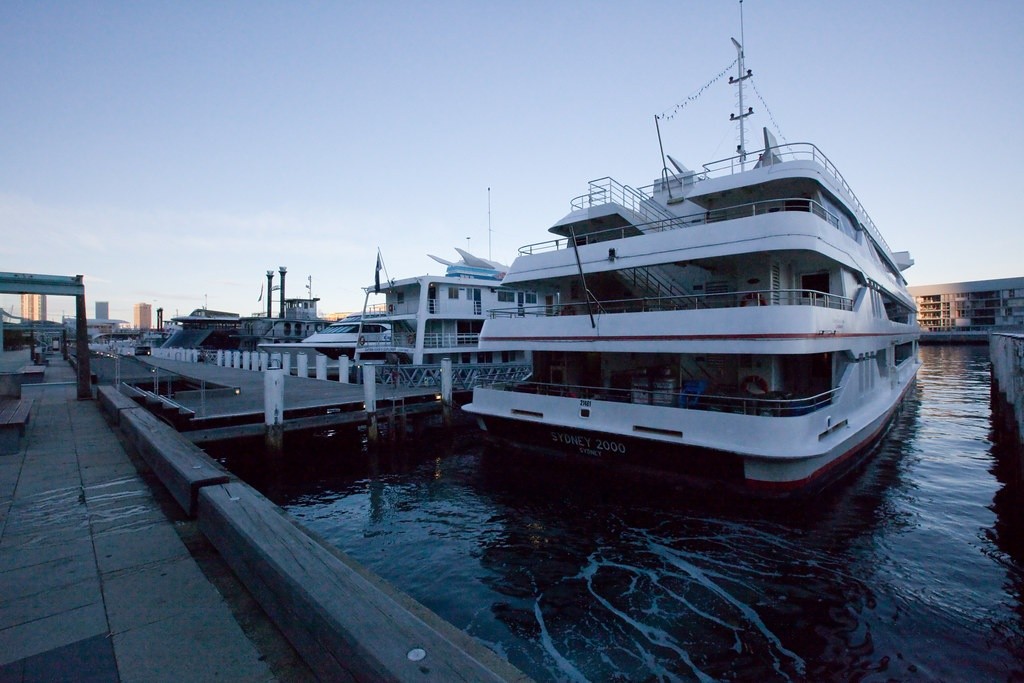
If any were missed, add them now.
[375,253,382,294]
[258,284,263,301]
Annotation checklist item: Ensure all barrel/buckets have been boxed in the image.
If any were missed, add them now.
[679,379,707,409]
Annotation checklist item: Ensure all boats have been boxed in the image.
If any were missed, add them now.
[92,245,561,392]
[458,0,923,501]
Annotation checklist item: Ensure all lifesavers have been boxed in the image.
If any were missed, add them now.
[358,335,366,346]
[739,374,770,397]
[739,290,767,307]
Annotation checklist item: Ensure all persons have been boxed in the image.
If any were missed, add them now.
[5,342,24,351]
[244,320,254,335]
[199,348,207,361]
